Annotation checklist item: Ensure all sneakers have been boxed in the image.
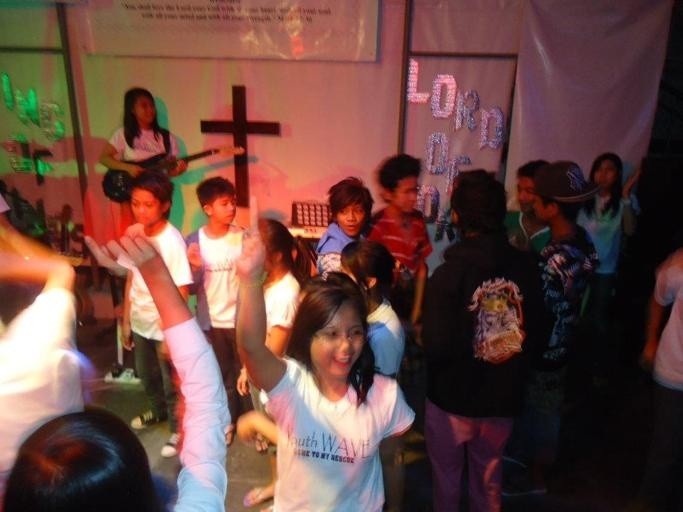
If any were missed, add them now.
[159,432,182,459]
[501,450,531,475]
[500,479,548,497]
[130,406,170,430]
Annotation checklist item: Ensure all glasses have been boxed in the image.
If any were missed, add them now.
[313,328,370,346]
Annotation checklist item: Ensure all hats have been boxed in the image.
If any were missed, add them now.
[534,160,604,201]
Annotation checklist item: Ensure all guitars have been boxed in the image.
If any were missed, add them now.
[55,204,94,329]
[102,145,245,202]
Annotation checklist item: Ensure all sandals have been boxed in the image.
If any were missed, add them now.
[222,421,235,447]
[255,435,269,454]
[242,484,274,509]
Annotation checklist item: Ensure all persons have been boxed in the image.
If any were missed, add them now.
[98,87,187,239]
[0,151,682,511]
